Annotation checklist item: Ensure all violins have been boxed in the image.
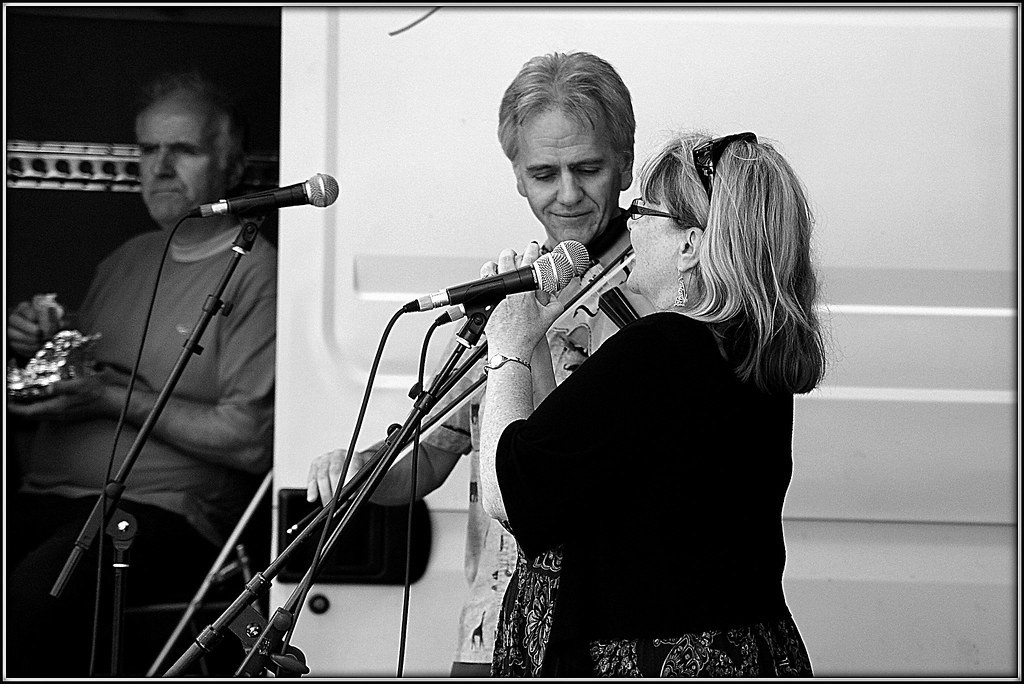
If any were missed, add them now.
[551,213,660,358]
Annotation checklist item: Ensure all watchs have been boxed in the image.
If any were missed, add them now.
[485,354,532,378]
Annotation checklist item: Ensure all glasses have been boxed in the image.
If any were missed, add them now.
[692,132,758,206]
[627,198,704,232]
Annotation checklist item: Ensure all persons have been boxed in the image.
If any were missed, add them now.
[479,132,826,678]
[6,75,275,677]
[307,52,659,678]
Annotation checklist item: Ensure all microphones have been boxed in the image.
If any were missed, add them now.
[187,174,339,218]
[404,252,573,314]
[435,241,590,326]
